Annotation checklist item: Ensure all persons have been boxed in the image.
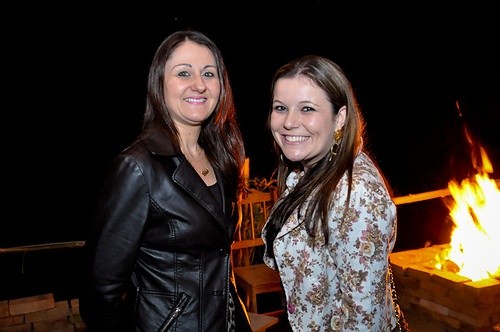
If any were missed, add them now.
[260,54,408,332]
[79,31,251,332]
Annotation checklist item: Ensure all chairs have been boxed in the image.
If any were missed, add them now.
[234,190,284,316]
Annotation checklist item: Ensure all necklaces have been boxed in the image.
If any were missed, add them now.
[181,148,209,176]
